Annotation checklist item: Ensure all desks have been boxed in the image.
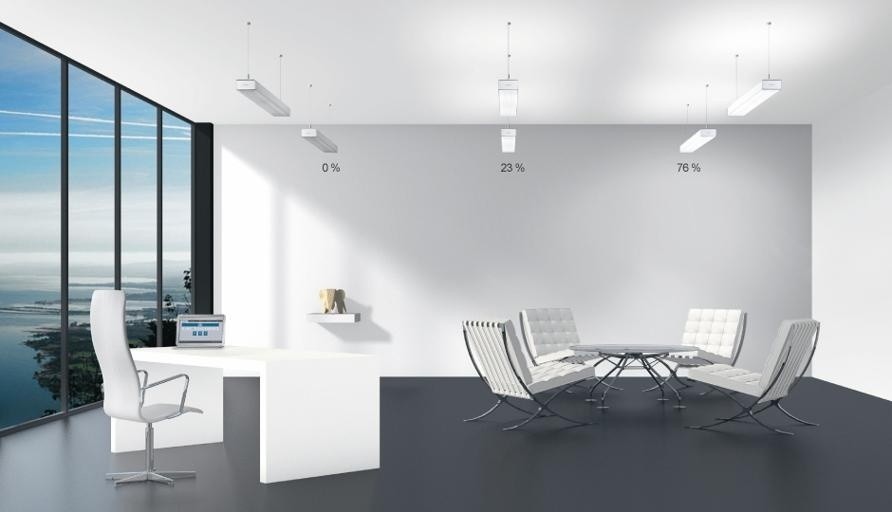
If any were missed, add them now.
[108,343,383,485]
[568,343,699,411]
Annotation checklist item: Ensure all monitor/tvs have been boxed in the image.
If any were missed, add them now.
[176,314,226,349]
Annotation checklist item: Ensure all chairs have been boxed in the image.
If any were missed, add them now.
[89,288,205,487]
[643,308,821,436]
[461,304,625,433]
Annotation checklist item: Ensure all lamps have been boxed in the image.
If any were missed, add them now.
[678,21,783,155]
[497,20,520,155]
[234,21,338,154]
